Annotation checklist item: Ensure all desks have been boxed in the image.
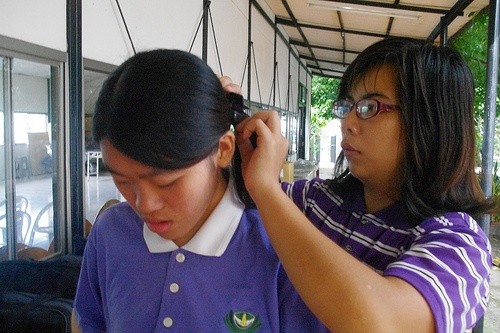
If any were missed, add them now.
[87,151,102,179]
[294,164,319,182]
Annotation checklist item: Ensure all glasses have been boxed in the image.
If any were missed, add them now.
[333,98,401,119]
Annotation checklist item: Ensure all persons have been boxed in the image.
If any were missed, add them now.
[237,38,500,333]
[72,49,334,333]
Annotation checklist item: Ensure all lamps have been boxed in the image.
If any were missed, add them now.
[307,0,423,22]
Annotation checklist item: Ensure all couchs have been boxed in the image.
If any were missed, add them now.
[0,259,79,333]
